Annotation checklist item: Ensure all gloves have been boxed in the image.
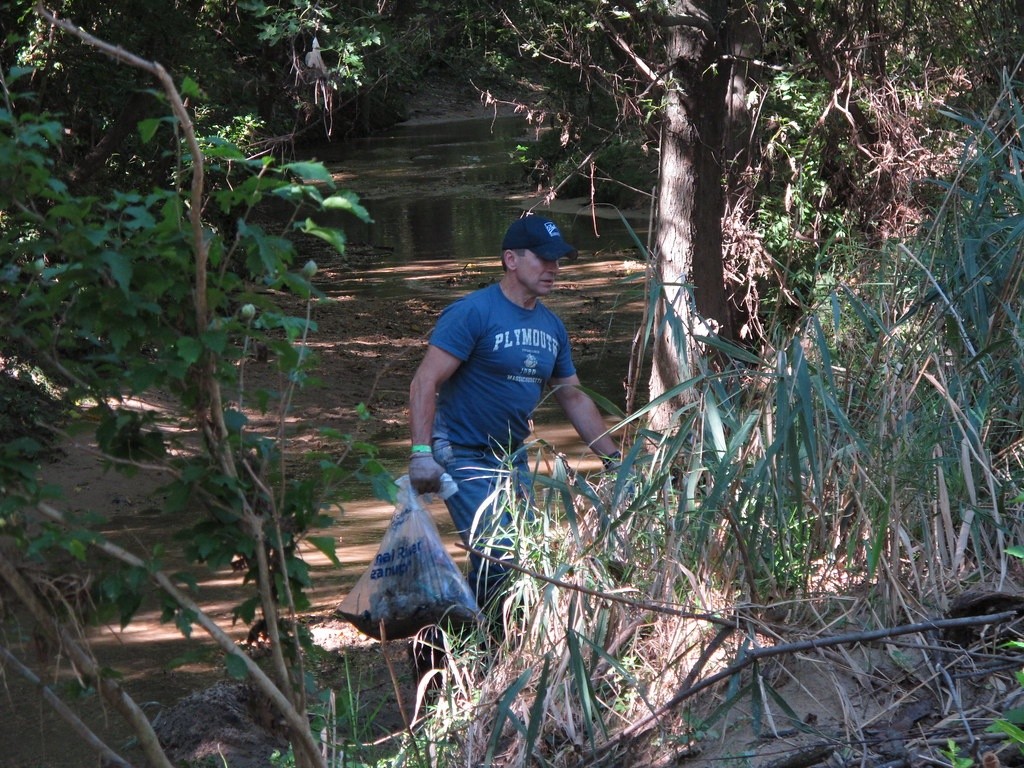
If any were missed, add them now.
[603,452,647,498]
[409,445,446,495]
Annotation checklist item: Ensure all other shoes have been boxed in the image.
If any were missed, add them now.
[407,641,445,710]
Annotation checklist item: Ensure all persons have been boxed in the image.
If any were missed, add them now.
[407,215,635,702]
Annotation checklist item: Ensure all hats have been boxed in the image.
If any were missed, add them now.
[502,215,578,261]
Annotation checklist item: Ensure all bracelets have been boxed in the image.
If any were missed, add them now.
[602,450,622,469]
[411,444,432,453]
